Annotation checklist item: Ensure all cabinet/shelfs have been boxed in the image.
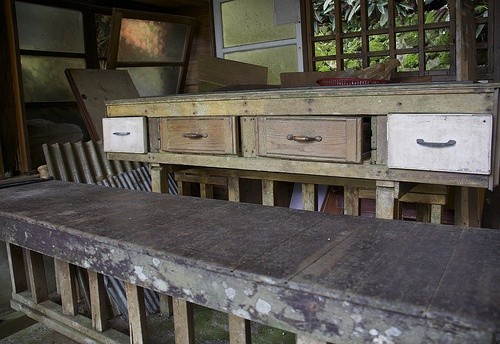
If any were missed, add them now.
[100,80,500,231]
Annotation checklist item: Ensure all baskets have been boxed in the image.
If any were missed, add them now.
[315,77,393,87]
